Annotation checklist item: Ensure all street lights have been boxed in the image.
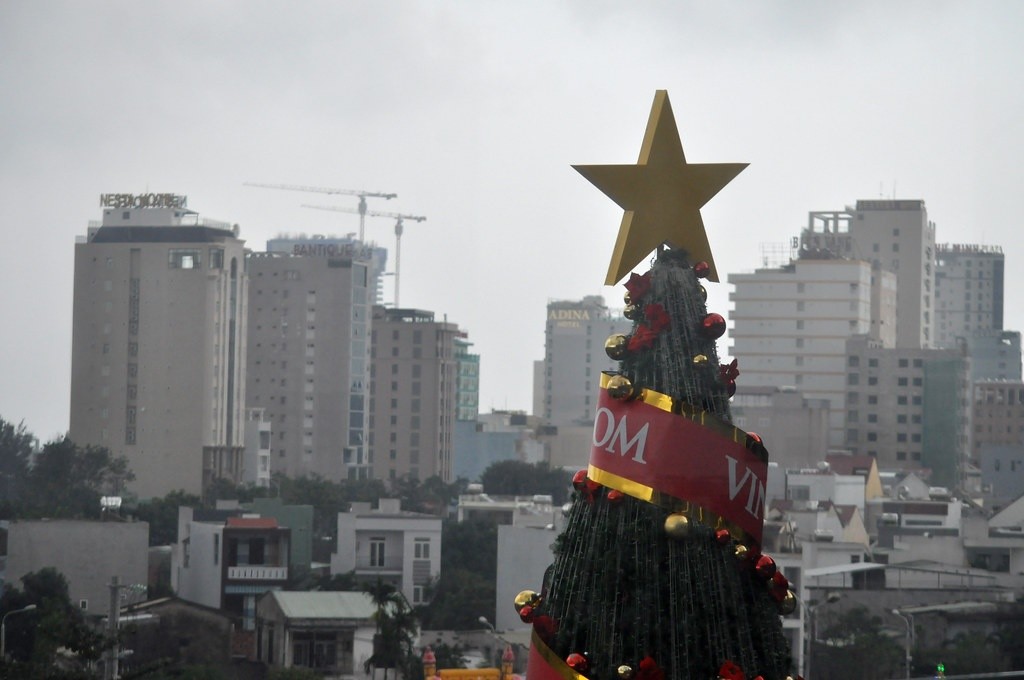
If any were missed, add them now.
[1,604,37,665]
[796,591,840,680]
[891,608,911,680]
[479,616,497,667]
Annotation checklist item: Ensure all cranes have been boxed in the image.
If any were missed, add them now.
[301,204,427,310]
[243,181,397,244]
